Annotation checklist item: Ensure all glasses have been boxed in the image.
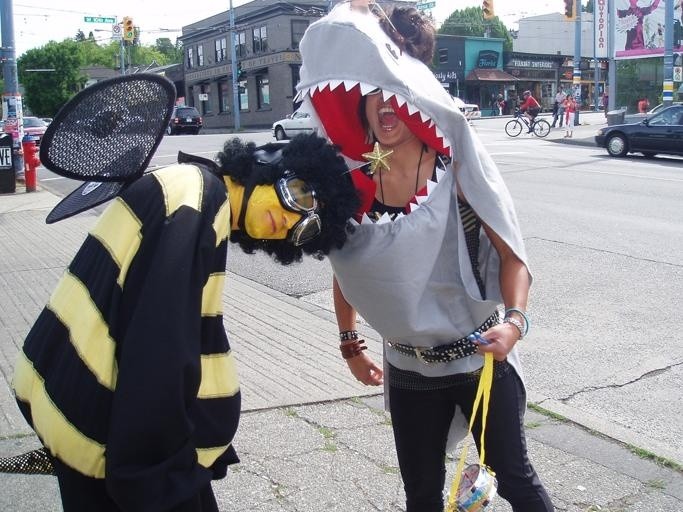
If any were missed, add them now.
[273,159,322,247]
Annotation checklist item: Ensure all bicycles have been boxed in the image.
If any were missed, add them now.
[502,106,550,139]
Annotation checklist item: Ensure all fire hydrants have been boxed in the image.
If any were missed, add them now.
[21,131,42,194]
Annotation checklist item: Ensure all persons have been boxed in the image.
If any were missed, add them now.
[10,132,362,512]
[488,85,577,139]
[292,1,555,511]
[638,96,651,113]
[602,91,609,119]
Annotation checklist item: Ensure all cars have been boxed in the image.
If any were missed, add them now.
[594,103,682,162]
[269,106,324,143]
[0,116,57,145]
[445,92,481,122]
[634,101,682,114]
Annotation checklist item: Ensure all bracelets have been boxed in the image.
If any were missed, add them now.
[503,307,531,340]
[337,331,367,359]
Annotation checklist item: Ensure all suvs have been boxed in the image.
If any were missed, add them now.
[148,104,203,135]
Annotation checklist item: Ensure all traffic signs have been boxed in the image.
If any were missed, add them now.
[415,1,435,9]
[83,15,115,25]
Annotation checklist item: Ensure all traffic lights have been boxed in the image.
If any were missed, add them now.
[563,0,577,22]
[481,0,494,20]
[122,17,134,41]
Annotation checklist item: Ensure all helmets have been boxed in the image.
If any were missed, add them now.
[523,90,531,96]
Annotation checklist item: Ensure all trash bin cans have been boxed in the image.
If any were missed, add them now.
[606,110,625,125]
[1,134,15,192]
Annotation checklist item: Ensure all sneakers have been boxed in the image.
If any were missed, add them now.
[526,118,535,135]
